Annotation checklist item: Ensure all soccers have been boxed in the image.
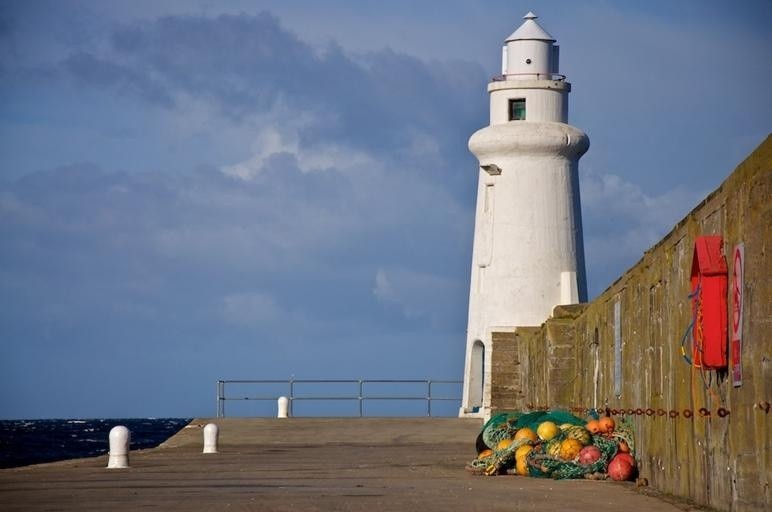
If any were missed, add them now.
[477,416,635,481]
